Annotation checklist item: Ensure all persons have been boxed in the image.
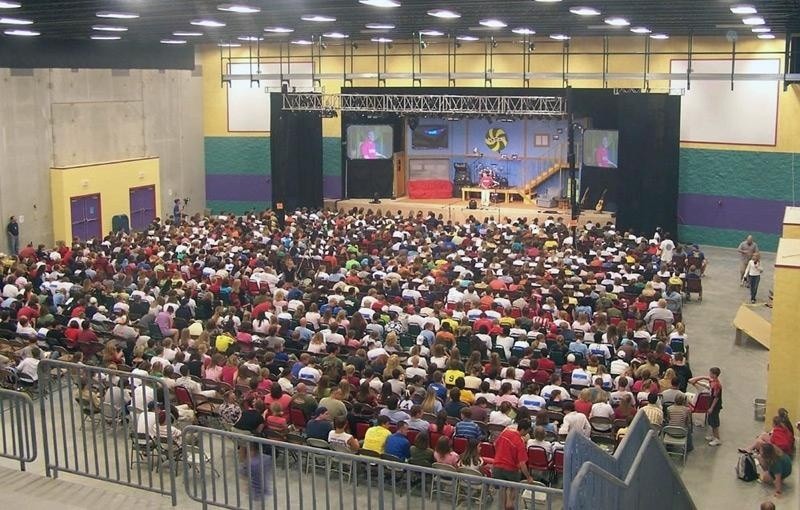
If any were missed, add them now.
[736,234,759,288]
[479,172,493,210]
[738,406,795,497]
[761,501,775,510]
[596,136,617,168]
[743,253,763,303]
[360,130,387,159]
[1,199,725,510]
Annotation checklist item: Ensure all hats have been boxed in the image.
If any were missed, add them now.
[119,314,128,324]
[395,296,403,302]
[160,410,166,416]
[148,401,163,408]
[90,297,97,304]
[566,353,576,363]
[98,306,109,313]
[617,351,626,358]
[446,308,453,315]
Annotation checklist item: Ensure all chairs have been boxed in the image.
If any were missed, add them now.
[1,202,724,509]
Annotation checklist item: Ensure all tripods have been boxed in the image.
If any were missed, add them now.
[296,249,314,276]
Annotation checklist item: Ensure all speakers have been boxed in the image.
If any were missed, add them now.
[469,200,476,209]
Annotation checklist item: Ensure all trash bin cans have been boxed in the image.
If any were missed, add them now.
[112,214,129,235]
[755,399,767,421]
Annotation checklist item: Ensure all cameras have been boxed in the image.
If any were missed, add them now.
[184,198,189,204]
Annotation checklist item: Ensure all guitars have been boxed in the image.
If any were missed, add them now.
[595,188,608,211]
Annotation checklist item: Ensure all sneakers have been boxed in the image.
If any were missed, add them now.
[738,448,753,455]
[705,435,721,446]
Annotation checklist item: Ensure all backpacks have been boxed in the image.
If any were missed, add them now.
[736,453,757,481]
